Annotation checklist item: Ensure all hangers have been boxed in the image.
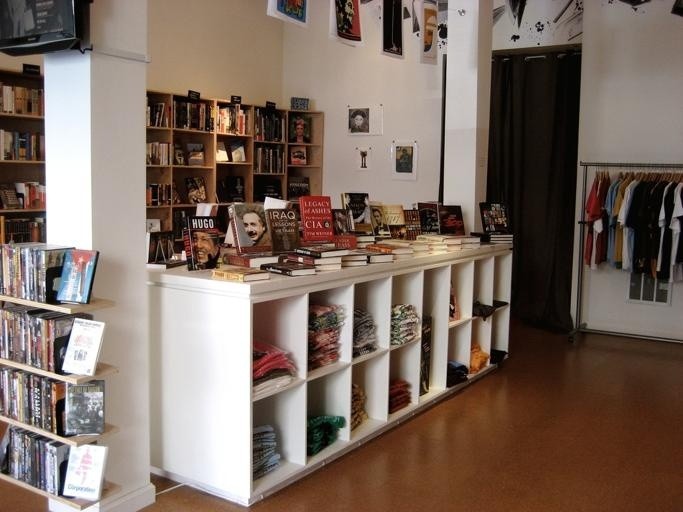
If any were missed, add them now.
[594,160,683,185]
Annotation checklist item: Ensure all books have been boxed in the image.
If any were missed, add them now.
[148,193,513,282]
[148,102,312,236]
[2,87,46,241]
[1,239,108,501]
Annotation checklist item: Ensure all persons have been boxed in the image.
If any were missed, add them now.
[8,0,27,38]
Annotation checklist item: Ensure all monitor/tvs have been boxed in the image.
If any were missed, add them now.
[0,0,91,57]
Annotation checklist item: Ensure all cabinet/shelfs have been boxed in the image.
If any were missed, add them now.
[0,291,120,512]
[145,236,513,508]
[145,90,324,233]
[0,68,45,244]
